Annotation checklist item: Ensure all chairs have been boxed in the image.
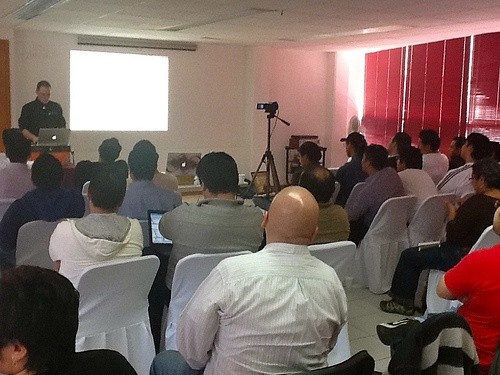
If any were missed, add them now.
[0,166,500,374]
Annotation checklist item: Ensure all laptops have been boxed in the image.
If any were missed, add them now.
[165,153,201,176]
[30,128,70,147]
[147,209,173,255]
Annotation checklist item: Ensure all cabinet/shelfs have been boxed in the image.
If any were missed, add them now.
[285,146,327,186]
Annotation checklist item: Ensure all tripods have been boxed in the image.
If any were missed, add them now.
[243,114,290,200]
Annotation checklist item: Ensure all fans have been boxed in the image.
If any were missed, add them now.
[347,115,367,135]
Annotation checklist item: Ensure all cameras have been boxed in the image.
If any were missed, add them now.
[257,102,278,114]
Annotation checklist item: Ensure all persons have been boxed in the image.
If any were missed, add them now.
[17,80,67,144]
[376,207,500,375]
[142,150,264,356]
[127,140,178,193]
[291,141,336,204]
[333,132,368,208]
[395,147,438,228]
[299,165,351,246]
[114,138,182,247]
[379,158,500,317]
[434,132,491,193]
[0,127,37,199]
[418,129,449,186]
[0,152,86,259]
[48,169,144,291]
[76,137,129,196]
[344,143,405,243]
[386,132,412,158]
[148,186,348,375]
[447,137,467,171]
[0,265,137,375]
